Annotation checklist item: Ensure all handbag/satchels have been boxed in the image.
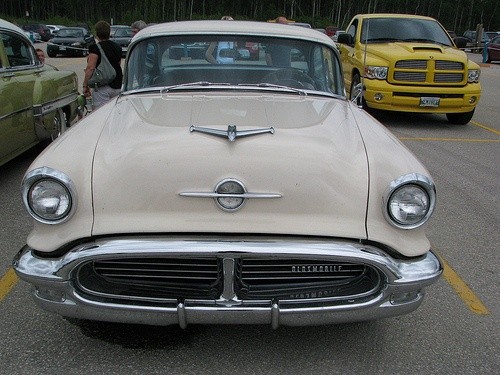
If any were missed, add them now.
[87,43,116,88]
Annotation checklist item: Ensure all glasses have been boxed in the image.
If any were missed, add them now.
[39,55,44,57]
[133,31,137,33]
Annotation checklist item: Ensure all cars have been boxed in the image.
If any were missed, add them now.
[109,26,131,56]
[446,30,500,54]
[481,35,500,63]
[11,20,444,330]
[20,22,65,43]
[169,20,346,60]
[109,25,129,37]
[322,14,481,126]
[47,26,96,58]
[0,18,87,165]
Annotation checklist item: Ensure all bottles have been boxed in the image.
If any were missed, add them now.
[86,97,92,111]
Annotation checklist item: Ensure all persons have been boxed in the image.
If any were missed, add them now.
[32,48,45,64]
[265,17,295,69]
[130,19,163,85]
[205,16,238,65]
[82,20,124,113]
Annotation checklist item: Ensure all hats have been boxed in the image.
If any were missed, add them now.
[221,16,233,21]
[276,17,288,24]
